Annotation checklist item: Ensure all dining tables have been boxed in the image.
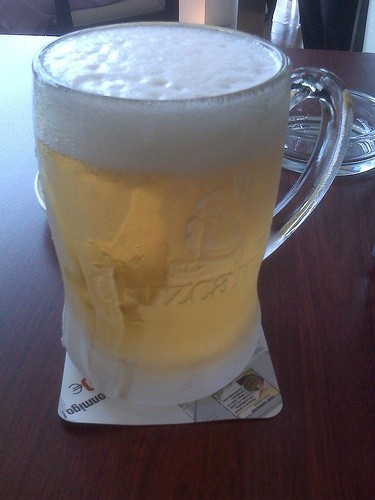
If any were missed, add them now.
[1,33,375,500]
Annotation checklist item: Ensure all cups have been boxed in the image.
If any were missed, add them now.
[31,22,353,409]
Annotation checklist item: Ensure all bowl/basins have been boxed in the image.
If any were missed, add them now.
[282,90,375,177]
